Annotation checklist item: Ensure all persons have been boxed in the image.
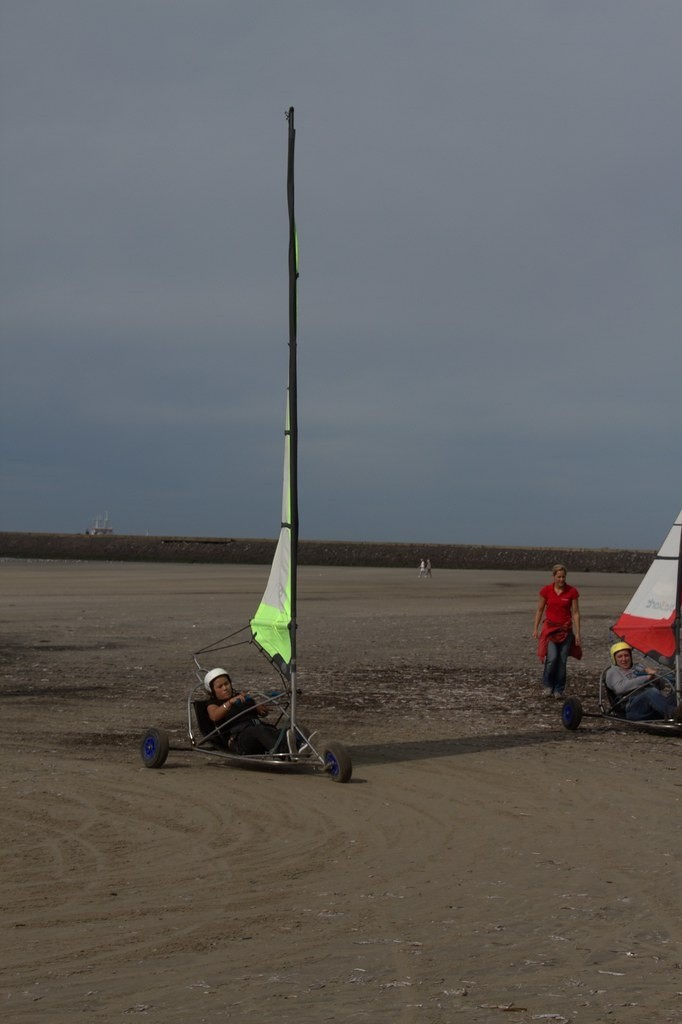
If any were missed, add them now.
[416,558,432,578]
[604,642,682,723]
[532,565,582,700]
[204,667,313,762]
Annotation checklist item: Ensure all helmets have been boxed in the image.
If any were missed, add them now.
[203,668,229,693]
[609,641,633,665]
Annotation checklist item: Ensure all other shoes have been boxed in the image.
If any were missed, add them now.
[674,702,682,723]
[298,731,321,755]
[286,729,298,760]
[544,687,553,696]
[554,691,565,700]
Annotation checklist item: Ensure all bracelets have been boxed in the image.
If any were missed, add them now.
[222,703,230,711]
[228,699,233,705]
